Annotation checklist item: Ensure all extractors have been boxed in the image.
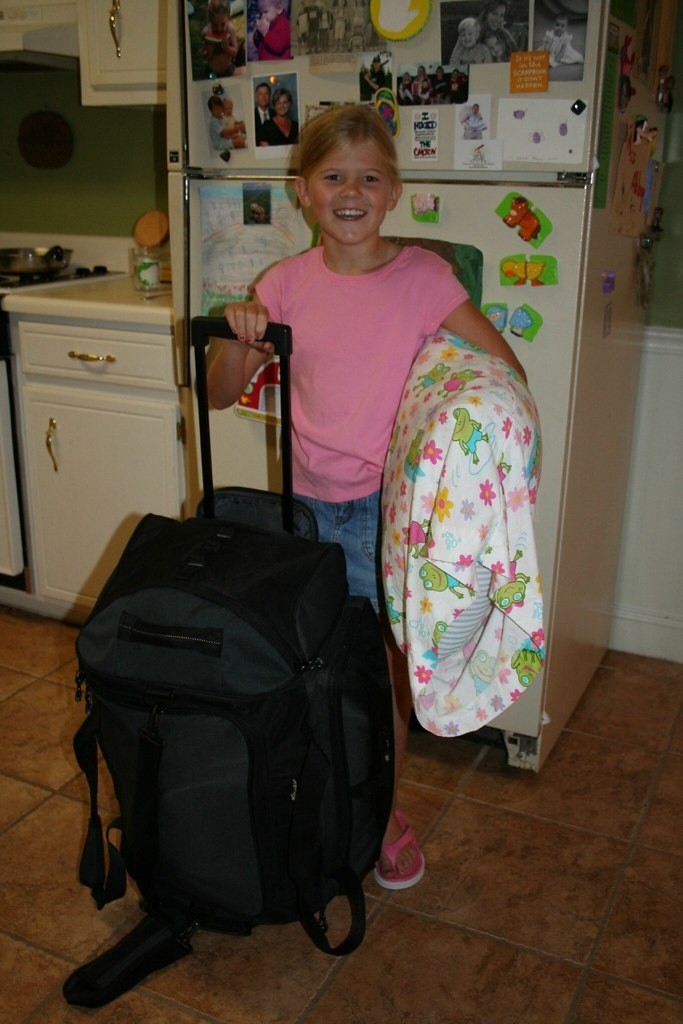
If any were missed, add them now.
[0,20,80,73]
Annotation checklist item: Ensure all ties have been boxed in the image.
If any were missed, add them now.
[264,113,268,121]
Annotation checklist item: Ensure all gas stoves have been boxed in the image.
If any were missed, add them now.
[0,264,125,288]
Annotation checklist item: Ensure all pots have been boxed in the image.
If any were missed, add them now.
[0,246,72,274]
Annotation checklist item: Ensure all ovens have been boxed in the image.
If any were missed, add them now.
[0,310,31,594]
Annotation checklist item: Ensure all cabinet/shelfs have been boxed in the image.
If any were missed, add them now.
[10,313,191,627]
[78,0,167,107]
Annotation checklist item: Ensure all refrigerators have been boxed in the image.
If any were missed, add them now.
[165,1,670,777]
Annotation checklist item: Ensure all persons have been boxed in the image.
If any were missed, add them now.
[460,104,487,139]
[248,0,293,60]
[535,14,583,67]
[449,0,520,65]
[206,95,247,151]
[205,104,527,890]
[364,54,385,101]
[201,0,238,80]
[255,82,298,147]
[398,66,466,104]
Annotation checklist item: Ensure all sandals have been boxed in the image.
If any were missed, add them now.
[374,809,424,889]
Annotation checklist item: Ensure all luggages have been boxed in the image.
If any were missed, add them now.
[65,314,397,1010]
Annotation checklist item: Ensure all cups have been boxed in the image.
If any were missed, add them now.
[127,244,159,290]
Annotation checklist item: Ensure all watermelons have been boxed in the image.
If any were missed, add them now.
[204,31,231,42]
